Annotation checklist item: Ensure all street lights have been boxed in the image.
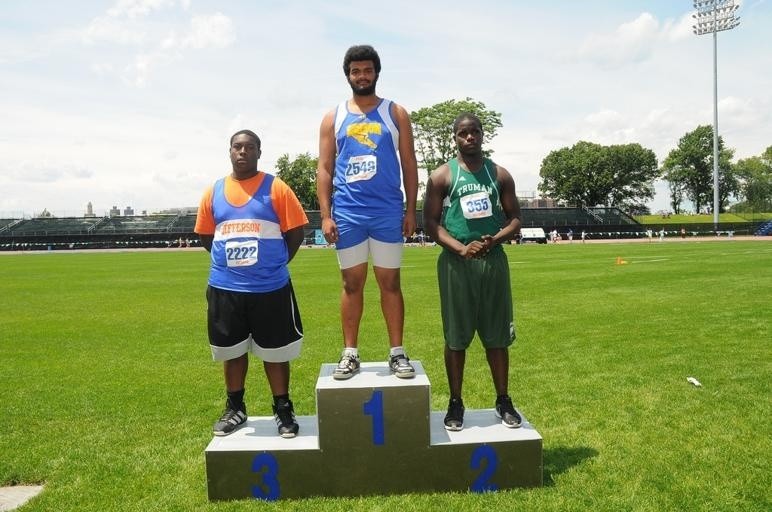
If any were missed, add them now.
[692,0,743,231]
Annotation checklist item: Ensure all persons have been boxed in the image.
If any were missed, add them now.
[193,129,309,438]
[317,44,419,379]
[177,236,191,248]
[646,226,686,241]
[422,112,523,431]
[401,227,589,246]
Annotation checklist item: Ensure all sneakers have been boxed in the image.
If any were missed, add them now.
[212,403,248,437]
[388,346,416,379]
[332,347,360,380]
[443,399,466,431]
[494,396,522,428]
[272,398,299,438]
